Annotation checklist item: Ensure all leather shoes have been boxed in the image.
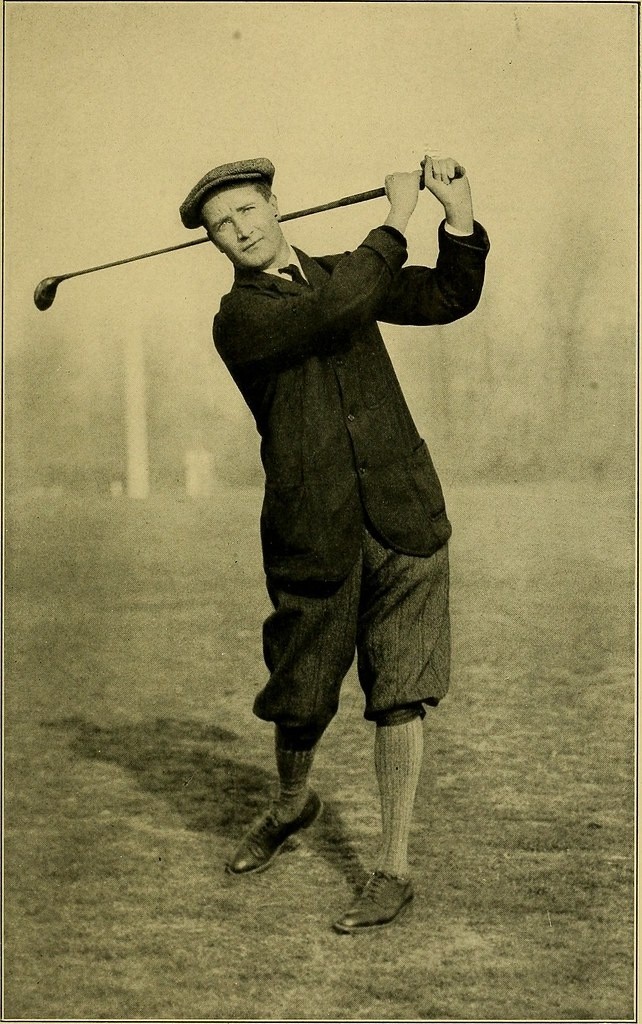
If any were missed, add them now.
[227,788,323,877]
[334,873,415,932]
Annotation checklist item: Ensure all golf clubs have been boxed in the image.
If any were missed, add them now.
[33,164,467,312]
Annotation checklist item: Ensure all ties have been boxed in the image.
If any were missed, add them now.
[278,265,310,287]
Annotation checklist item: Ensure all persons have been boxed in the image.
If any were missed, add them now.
[176,158,495,931]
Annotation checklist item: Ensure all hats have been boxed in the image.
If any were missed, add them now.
[179,158,275,229]
